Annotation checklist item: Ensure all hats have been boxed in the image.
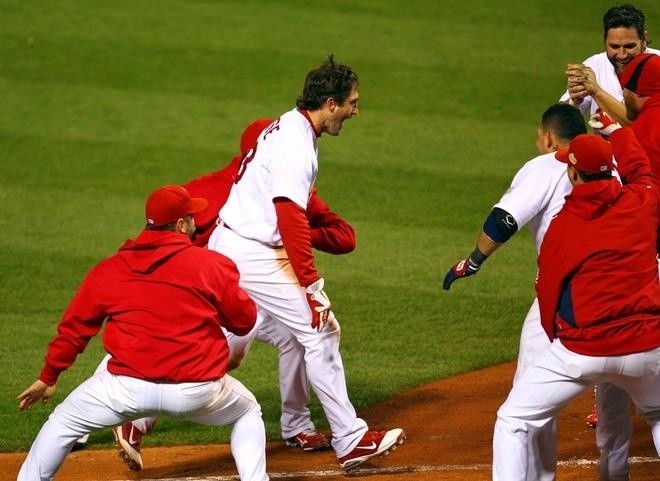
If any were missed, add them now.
[621,53,660,95]
[146,186,208,226]
[556,135,614,173]
[241,120,274,154]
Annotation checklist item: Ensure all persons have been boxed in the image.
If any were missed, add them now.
[19,184,272,480]
[205,52,407,470]
[111,117,356,472]
[556,4,659,138]
[441,56,660,481]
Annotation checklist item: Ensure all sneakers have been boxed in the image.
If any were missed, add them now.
[584,410,598,426]
[338,428,406,470]
[285,430,329,451]
[112,422,144,471]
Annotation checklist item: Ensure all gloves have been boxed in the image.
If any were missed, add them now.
[588,110,622,136]
[443,255,480,290]
[306,278,332,333]
